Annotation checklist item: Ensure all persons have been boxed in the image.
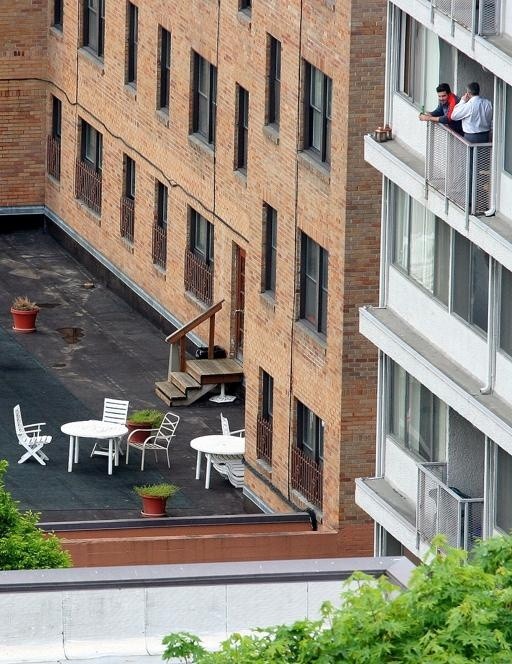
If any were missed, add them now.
[418,82,492,207]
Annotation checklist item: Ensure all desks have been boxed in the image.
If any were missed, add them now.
[60,419,129,476]
[189,435,246,490]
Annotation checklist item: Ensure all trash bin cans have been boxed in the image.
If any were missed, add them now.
[195,346,226,359]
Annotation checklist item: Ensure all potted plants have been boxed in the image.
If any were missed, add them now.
[10,296,40,334]
[134,483,181,518]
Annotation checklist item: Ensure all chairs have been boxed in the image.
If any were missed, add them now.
[88,397,181,472]
[13,403,53,466]
